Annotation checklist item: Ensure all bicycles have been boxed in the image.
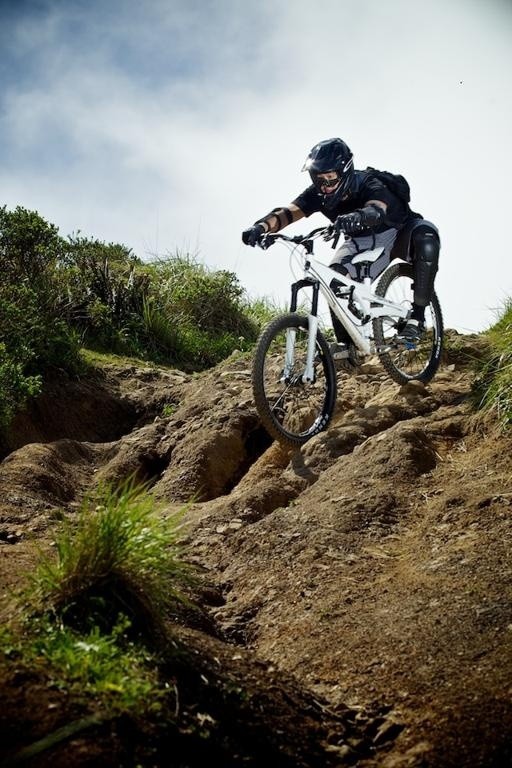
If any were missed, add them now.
[251,219,444,449]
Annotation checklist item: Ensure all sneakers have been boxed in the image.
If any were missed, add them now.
[394,317,426,341]
[330,342,352,360]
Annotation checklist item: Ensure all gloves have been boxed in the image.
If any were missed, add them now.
[241,227,262,249]
[336,211,363,235]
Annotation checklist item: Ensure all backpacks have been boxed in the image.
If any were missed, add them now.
[362,165,412,206]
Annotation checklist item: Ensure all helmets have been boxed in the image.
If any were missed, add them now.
[300,136,354,210]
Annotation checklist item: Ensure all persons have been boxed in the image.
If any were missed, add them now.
[241,137,442,365]
